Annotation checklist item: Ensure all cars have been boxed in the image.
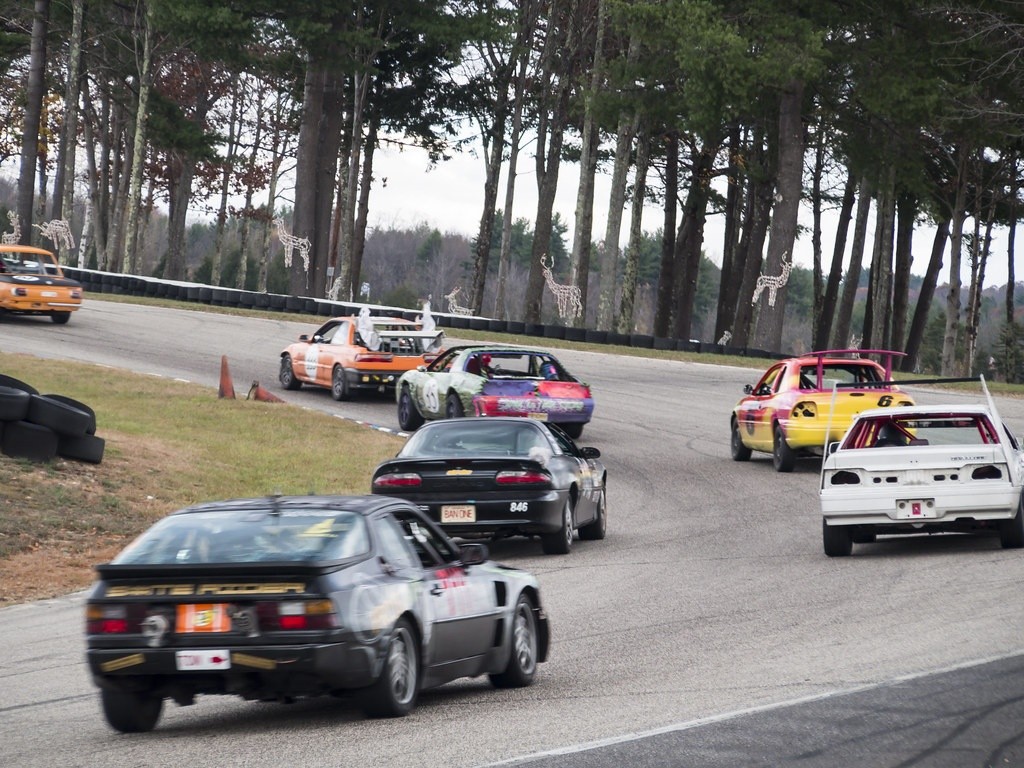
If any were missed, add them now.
[0,244,84,324]
[818,374,1024,556]
[730,349,918,473]
[396,343,594,439]
[371,416,608,555]
[85,495,552,732]
[279,316,453,401]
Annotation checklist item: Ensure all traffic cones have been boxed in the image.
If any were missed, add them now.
[218,355,236,399]
[246,380,286,403]
